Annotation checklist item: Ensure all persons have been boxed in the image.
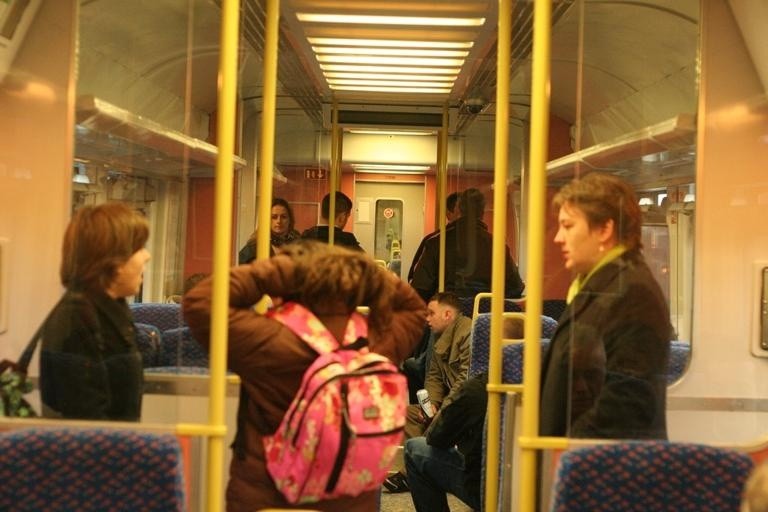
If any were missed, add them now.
[404,317,523,512]
[539,172,672,439]
[381,294,473,493]
[408,192,461,285]
[181,240,426,512]
[238,197,298,264]
[302,191,365,254]
[40,200,151,422]
[413,188,524,298]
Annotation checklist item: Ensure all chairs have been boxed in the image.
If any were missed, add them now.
[127,302,209,374]
[0,424,191,511]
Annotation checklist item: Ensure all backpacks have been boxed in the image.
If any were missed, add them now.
[263,300,410,507]
[0,317,50,418]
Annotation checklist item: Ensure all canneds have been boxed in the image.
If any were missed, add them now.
[417,388,434,419]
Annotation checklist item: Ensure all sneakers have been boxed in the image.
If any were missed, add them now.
[384,468,411,493]
